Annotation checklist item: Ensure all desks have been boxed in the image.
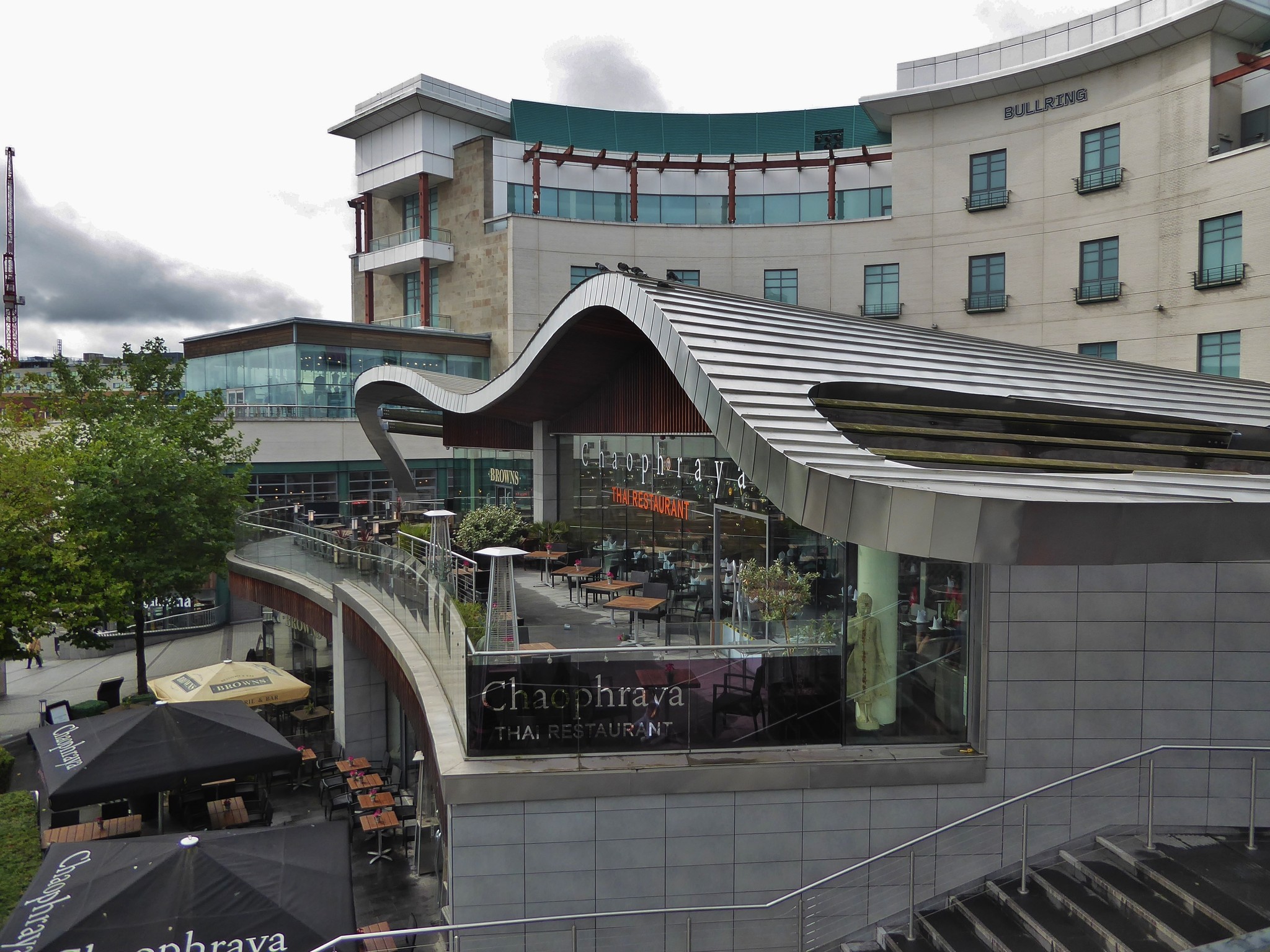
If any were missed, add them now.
[420,552,966,751]
[201,697,398,865]
[317,511,427,541]
[45,814,142,846]
[356,920,397,952]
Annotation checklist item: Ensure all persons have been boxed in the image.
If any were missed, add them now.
[847,592,889,722]
[25,632,43,669]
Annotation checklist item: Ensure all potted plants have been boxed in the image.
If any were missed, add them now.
[393,498,404,520]
[356,527,377,576]
[304,702,316,714]
[335,529,351,567]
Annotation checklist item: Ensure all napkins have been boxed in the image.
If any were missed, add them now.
[916,609,927,622]
[842,585,852,595]
[851,589,857,601]
[947,576,956,587]
[601,538,840,586]
[957,610,967,622]
[932,616,943,629]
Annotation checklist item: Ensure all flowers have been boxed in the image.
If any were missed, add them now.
[350,769,357,776]
[463,561,469,566]
[369,790,376,797]
[358,772,364,778]
[374,809,381,817]
[575,559,582,565]
[607,572,614,579]
[223,798,232,805]
[348,756,353,761]
[546,545,552,550]
[95,817,102,824]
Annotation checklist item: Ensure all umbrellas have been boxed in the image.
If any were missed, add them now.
[0,819,358,952]
[25,701,301,835]
[146,660,311,707]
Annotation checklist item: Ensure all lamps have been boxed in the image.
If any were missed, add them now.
[294,503,379,534]
[39,699,47,713]
[385,500,390,510]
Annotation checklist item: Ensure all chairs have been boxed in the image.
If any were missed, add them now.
[169,740,416,857]
[397,912,417,952]
[49,810,79,829]
[102,801,129,820]
[451,537,701,652]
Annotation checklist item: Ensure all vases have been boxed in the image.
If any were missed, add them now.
[375,817,380,822]
[97,824,103,829]
[547,550,552,554]
[465,566,468,571]
[576,565,581,571]
[359,778,364,783]
[225,805,231,811]
[349,761,353,766]
[608,578,613,584]
[352,776,356,780]
[371,797,376,801]
